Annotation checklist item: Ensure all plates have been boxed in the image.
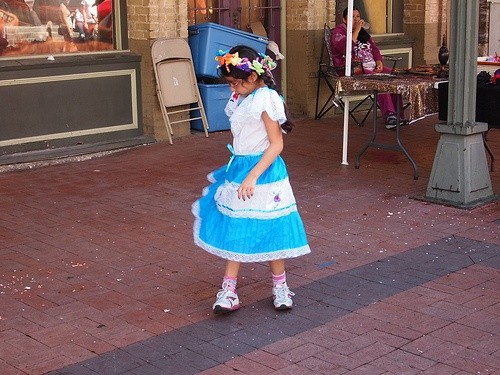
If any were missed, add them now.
[409,69,435,75]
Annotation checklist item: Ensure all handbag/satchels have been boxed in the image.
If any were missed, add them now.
[58,28,67,35]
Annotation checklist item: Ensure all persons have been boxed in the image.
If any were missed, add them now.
[192,45,312,314]
[56,0,113,44]
[330,6,408,128]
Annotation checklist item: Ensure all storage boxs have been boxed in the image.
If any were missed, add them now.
[190,83,234,132]
[189,22,269,83]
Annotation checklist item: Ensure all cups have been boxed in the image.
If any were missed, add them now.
[358,19,370,29]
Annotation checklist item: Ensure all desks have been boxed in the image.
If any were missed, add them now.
[333,64,500,180]
[439,82,500,172]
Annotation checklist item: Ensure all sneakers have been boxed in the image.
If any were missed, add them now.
[385,117,409,129]
[212,281,239,314]
[272,283,296,310]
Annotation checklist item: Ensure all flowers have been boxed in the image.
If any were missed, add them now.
[214,47,277,76]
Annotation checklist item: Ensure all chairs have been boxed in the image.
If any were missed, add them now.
[315,23,403,127]
[151,37,209,144]
